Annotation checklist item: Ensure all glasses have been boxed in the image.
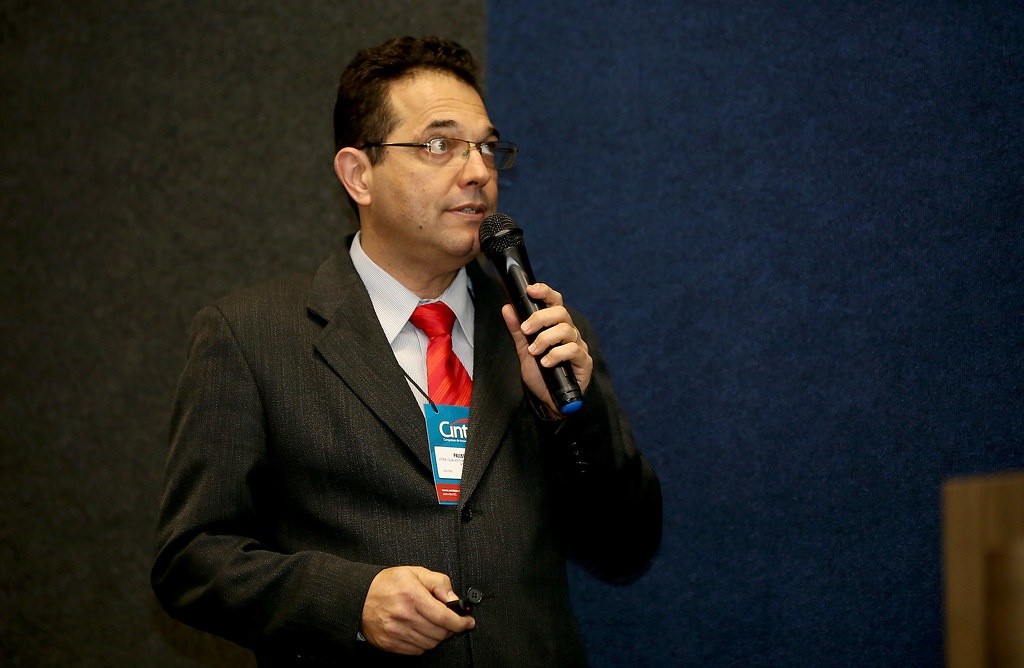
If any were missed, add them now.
[358,137,519,170]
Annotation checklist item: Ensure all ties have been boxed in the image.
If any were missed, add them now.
[409,301,472,407]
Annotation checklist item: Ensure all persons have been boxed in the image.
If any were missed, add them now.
[150,37,663,667]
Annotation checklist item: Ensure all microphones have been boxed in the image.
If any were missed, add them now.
[478,212,584,414]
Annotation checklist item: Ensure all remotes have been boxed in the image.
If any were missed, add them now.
[445,599,473,616]
